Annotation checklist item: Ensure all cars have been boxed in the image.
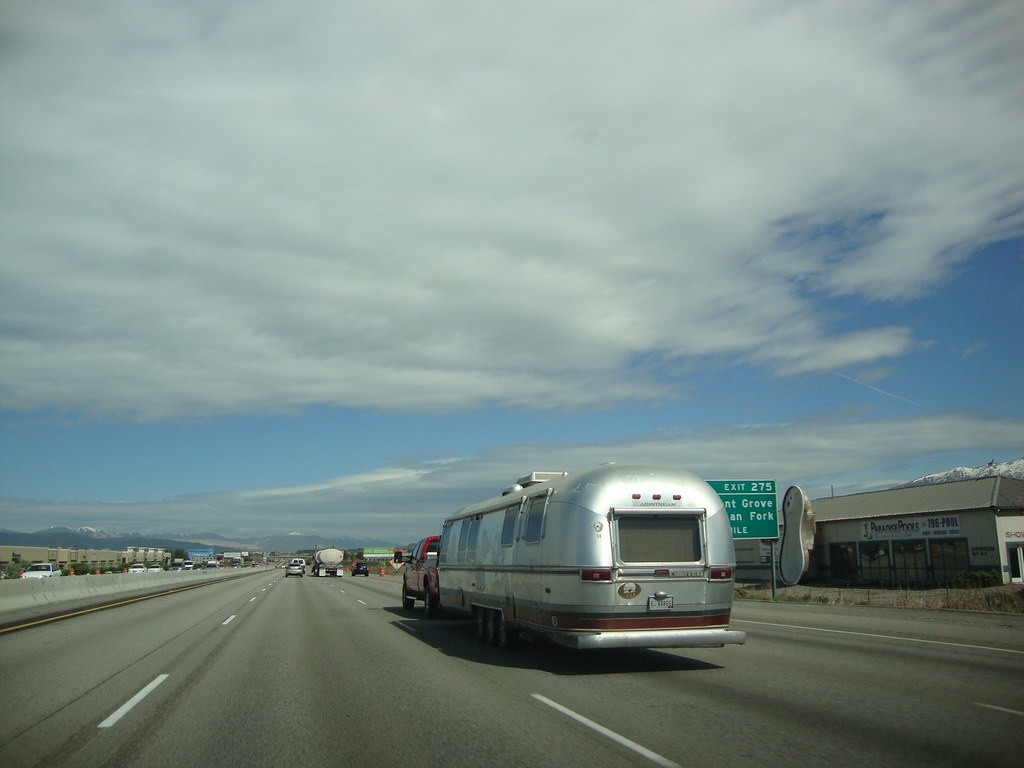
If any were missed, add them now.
[19,562,62,580]
[284,563,305,578]
[147,564,163,572]
[127,563,147,573]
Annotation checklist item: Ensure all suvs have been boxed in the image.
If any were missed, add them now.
[351,561,369,577]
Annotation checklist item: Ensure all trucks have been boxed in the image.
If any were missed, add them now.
[205,560,217,568]
[291,558,306,574]
[183,560,194,571]
[311,547,345,578]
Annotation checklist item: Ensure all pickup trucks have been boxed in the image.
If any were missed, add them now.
[393,531,443,620]
